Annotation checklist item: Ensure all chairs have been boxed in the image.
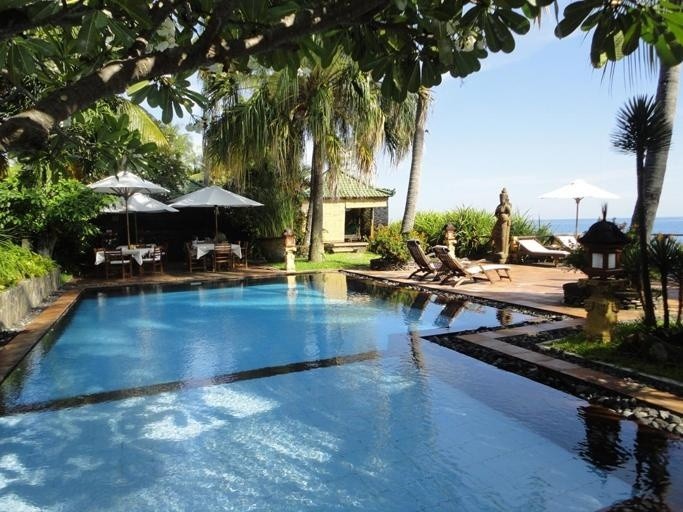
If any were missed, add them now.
[94,243,167,279]
[406,239,511,288]
[185,239,249,273]
[515,234,580,268]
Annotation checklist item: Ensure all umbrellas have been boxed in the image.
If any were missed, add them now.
[99,192,179,244]
[536,176,620,234]
[86,170,170,248]
[168,186,265,240]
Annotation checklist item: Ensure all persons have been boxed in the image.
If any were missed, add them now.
[491,187,512,256]
